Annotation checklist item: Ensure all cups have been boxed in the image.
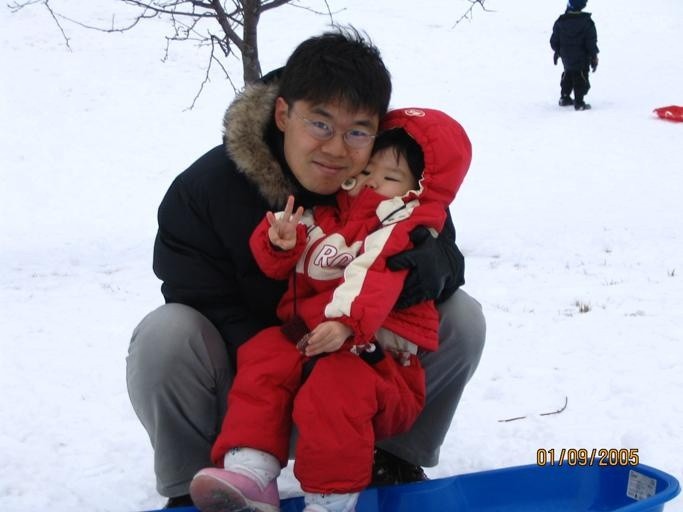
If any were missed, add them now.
[289,105,376,148]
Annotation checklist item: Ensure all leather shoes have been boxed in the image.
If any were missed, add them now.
[590,56,599,72]
[386,223,454,310]
[554,54,559,65]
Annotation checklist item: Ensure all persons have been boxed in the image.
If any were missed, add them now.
[549,0,599,110]
[125,22,486,510]
[190,106,471,512]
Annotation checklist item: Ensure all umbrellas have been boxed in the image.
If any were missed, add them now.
[370,449,428,487]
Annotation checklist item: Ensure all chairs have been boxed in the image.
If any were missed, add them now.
[190,467,280,510]
[559,97,591,110]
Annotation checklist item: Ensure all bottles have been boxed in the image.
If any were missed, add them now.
[565,0,588,11]
